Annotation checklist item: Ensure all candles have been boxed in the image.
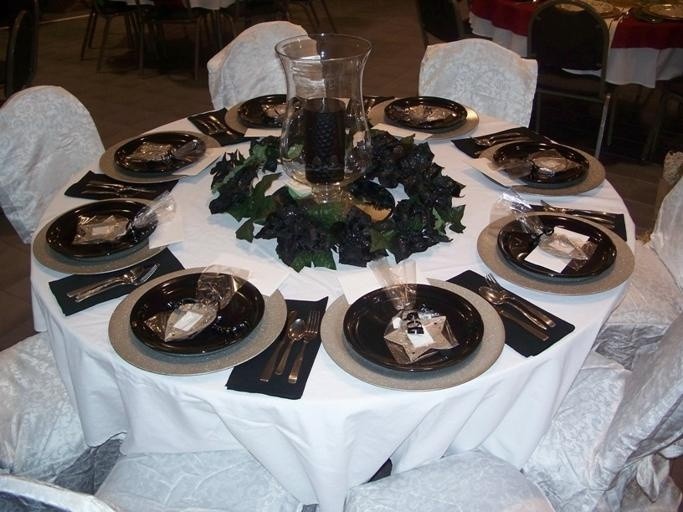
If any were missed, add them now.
[307,99,345,183]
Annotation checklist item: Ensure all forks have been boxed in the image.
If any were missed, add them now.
[287,311,321,384]
[196,118,219,135]
[541,198,615,218]
[485,273,557,328]
[74,263,161,303]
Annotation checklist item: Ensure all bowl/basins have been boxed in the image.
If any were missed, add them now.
[114,132,207,177]
[498,215,617,281]
[384,97,467,134]
[45,200,158,262]
[343,283,485,373]
[238,94,287,129]
[129,272,266,358]
[492,141,591,190]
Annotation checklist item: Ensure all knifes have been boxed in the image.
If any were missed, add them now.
[89,179,155,193]
[209,115,233,135]
[260,309,299,382]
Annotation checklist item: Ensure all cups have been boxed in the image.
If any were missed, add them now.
[274,32,372,222]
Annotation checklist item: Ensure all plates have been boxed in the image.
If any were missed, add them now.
[477,211,636,296]
[367,97,479,140]
[632,2,682,27]
[479,140,606,196]
[32,198,166,276]
[320,278,507,392]
[224,101,248,134]
[596,0,620,21]
[108,265,287,377]
[99,130,222,184]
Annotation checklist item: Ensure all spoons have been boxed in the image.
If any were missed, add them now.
[478,286,548,331]
[475,132,520,145]
[275,318,306,376]
[65,267,142,298]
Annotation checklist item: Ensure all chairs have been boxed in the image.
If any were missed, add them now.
[0,331,89,508]
[210,22,327,104]
[136,0,215,81]
[0,8,32,102]
[0,437,301,512]
[418,39,537,125]
[639,81,681,166]
[81,0,145,73]
[289,0,339,37]
[594,166,683,368]
[522,316,680,509]
[344,447,559,512]
[527,0,614,162]
[0,85,105,243]
[219,0,292,50]
[417,0,490,46]
